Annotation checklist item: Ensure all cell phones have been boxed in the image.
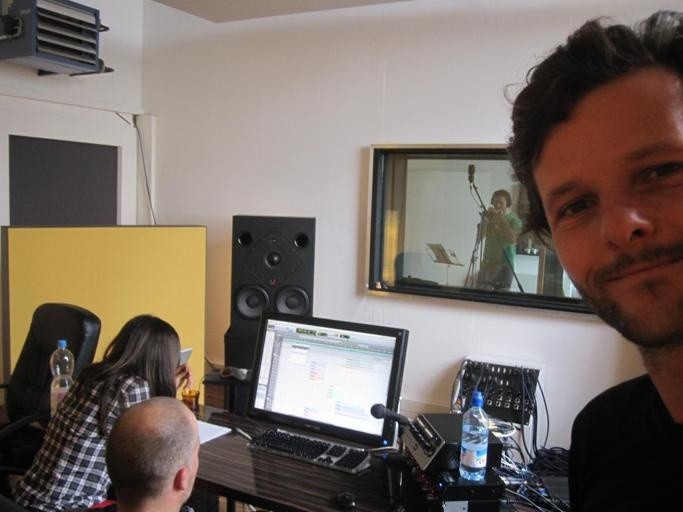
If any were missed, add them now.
[176,347,193,367]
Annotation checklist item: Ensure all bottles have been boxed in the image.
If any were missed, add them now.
[459,390,489,483]
[49,339,74,424]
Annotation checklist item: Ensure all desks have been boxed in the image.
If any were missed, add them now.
[177,363,572,512]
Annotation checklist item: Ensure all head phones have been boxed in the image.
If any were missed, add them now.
[491,189,512,208]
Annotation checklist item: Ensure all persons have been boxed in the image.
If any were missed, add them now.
[10,315,191,512]
[506,8,682,512]
[88,396,199,512]
[477,189,522,293]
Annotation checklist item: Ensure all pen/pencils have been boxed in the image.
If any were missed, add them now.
[235,427,253,441]
[184,387,196,397]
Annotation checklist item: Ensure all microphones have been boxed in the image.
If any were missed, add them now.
[468,164,475,183]
[370,403,409,427]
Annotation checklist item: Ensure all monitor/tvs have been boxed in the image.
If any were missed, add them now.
[246,310,410,449]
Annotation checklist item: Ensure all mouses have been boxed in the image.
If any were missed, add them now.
[336,492,357,509]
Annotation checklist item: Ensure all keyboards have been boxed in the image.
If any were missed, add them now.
[246,426,373,475]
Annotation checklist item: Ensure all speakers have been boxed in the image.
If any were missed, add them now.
[230,215,316,336]
